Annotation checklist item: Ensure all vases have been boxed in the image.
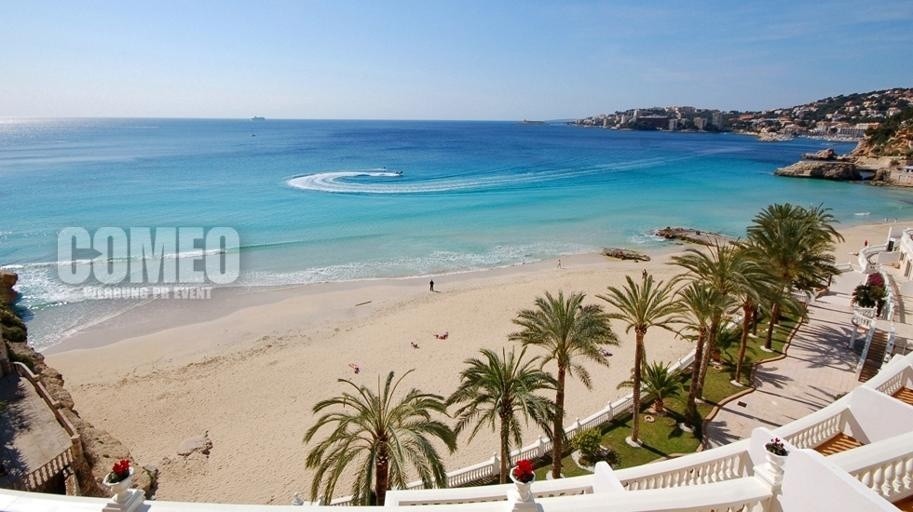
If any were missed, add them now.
[102,465,134,498]
[509,465,536,499]
[763,441,791,472]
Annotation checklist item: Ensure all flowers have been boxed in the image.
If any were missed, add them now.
[513,458,533,483]
[770,436,787,455]
[850,270,888,308]
[106,459,131,483]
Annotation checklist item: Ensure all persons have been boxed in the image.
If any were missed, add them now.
[433,331,448,339]
[430,280,433,291]
[557,259,561,269]
[349,362,360,371]
